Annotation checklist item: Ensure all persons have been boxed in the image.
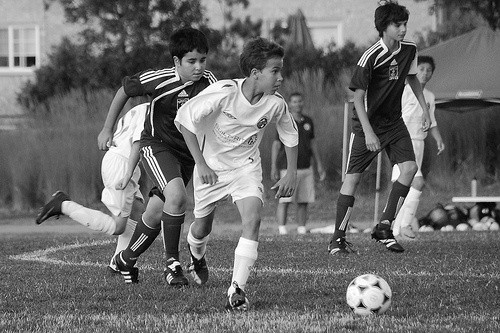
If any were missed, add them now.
[380,54,446,240]
[326,0,433,256]
[171,36,301,311]
[268,91,326,236]
[34,98,157,277]
[95,25,220,287]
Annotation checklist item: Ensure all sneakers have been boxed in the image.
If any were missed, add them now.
[188,243,208,285]
[371,224,404,253]
[112,250,139,285]
[225,281,250,312]
[106,266,120,277]
[36,190,70,224]
[328,237,354,255]
[164,256,188,288]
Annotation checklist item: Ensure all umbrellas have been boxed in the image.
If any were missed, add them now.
[283,7,316,71]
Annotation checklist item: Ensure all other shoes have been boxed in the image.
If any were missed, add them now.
[401,225,416,238]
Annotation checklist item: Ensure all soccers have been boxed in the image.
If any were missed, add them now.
[345,273,393,317]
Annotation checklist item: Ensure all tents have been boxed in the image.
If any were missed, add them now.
[341,25,500,231]
